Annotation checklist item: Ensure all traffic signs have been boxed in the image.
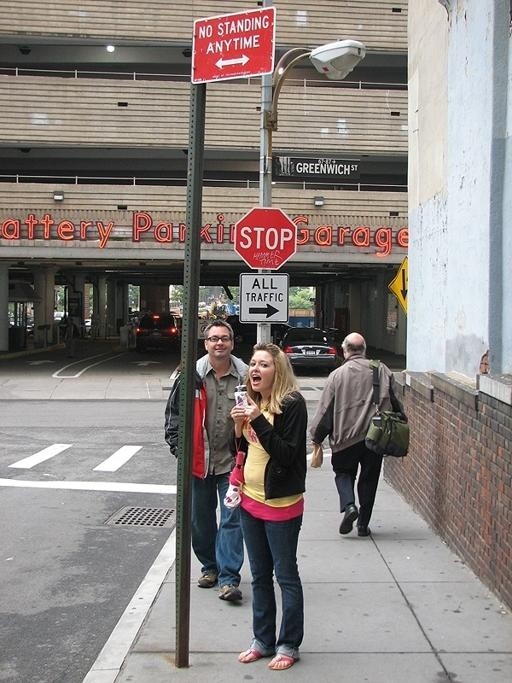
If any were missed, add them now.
[238,272,291,324]
[191,5,276,83]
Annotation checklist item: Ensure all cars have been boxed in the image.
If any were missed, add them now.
[84,319,92,335]
[225,314,295,348]
[278,326,338,375]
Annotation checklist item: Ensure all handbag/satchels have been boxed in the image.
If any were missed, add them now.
[365,410,410,456]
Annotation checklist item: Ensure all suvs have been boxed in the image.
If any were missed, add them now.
[136,310,181,352]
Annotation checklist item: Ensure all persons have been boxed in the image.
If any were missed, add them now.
[233,342,308,672]
[64,317,79,357]
[312,335,403,537]
[165,319,251,601]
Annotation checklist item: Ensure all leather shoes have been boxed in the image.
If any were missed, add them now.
[339,505,358,534]
[357,525,370,537]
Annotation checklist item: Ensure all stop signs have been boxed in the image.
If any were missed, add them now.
[234,207,298,271]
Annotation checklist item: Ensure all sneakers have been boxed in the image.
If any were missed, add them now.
[198,572,217,588]
[218,584,243,601]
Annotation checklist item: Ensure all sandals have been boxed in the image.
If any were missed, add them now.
[239,648,263,663]
[269,653,295,670]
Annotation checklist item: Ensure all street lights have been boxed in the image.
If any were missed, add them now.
[253,38,367,347]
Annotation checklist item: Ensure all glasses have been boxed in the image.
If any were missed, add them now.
[205,334,231,342]
